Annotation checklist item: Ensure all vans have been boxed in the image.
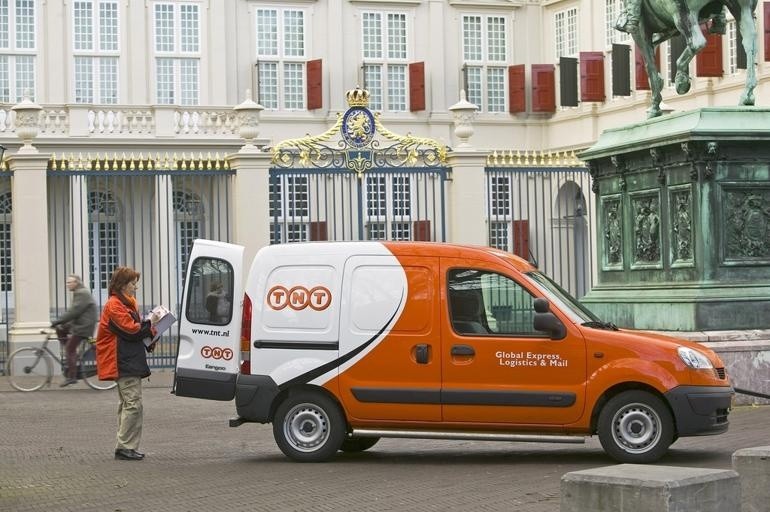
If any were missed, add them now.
[170,237,735,464]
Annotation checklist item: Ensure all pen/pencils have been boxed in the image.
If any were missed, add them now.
[149,311,153,313]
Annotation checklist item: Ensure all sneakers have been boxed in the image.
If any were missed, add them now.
[59,377,77,387]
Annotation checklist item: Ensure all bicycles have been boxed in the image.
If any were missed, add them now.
[3,325,119,394]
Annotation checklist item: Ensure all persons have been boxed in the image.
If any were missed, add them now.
[206,279,230,324]
[50,274,97,388]
[95,265,160,461]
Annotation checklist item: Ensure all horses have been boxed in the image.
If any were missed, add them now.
[622,0,758,120]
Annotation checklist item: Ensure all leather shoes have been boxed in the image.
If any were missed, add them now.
[115,449,145,460]
